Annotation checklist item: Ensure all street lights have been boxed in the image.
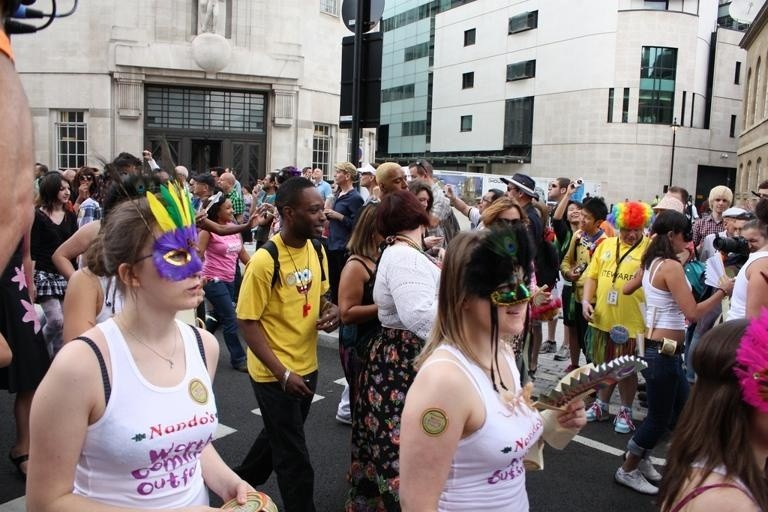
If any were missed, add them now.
[669,116,680,187]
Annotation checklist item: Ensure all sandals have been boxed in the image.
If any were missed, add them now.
[9,444,29,479]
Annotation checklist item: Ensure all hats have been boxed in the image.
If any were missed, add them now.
[653,194,685,215]
[358,163,376,176]
[193,172,215,187]
[722,207,750,219]
[335,162,357,181]
[500,172,539,202]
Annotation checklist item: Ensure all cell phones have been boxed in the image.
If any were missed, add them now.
[573,262,588,274]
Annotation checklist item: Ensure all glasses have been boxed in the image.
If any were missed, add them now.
[548,183,563,188]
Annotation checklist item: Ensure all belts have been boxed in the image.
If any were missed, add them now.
[646,338,685,353]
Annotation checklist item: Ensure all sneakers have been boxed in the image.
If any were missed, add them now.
[558,365,580,379]
[625,449,663,482]
[539,340,557,353]
[335,404,352,425]
[614,406,635,433]
[586,398,610,422]
[614,467,659,495]
[554,344,572,361]
[231,356,248,373]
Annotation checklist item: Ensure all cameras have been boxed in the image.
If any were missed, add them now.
[713,236,748,265]
[573,177,584,188]
